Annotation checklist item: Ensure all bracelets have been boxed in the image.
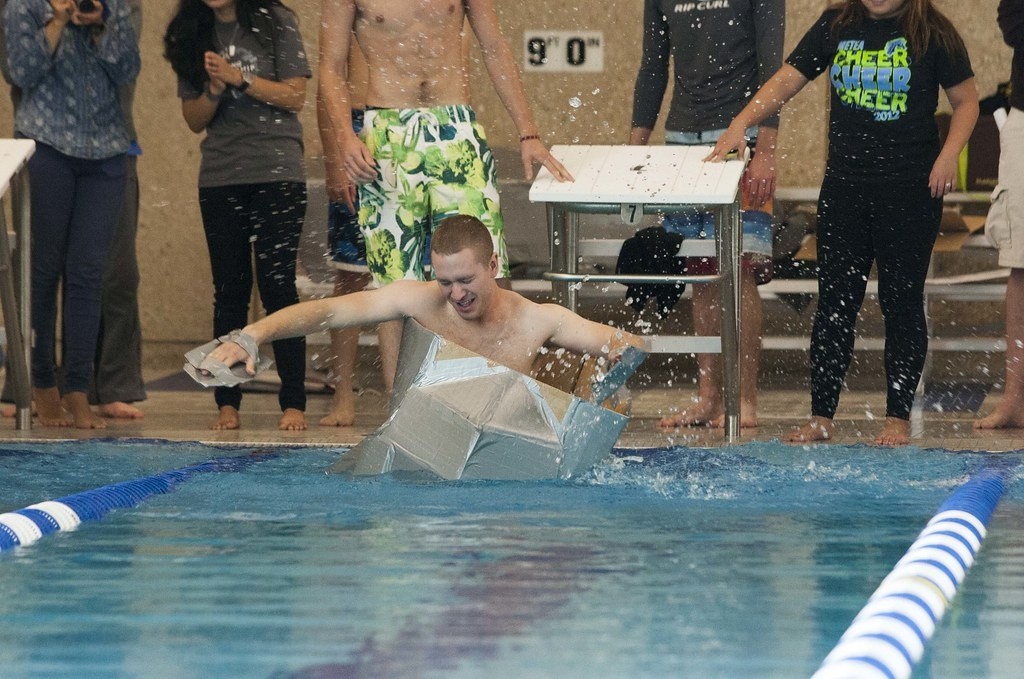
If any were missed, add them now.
[204,81,220,101]
[520,136,540,142]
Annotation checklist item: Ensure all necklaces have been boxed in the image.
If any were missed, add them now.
[213,21,239,52]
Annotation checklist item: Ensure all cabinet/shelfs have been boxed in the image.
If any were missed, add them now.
[242,177,1008,418]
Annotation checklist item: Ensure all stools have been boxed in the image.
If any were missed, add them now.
[526,141,753,446]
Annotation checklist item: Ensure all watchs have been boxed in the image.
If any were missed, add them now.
[238,76,252,93]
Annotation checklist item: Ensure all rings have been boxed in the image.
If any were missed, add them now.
[945,183,951,187]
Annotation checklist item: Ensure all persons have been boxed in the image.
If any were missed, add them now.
[162,0,313,431]
[974,0,1024,429]
[320,0,576,412]
[316,26,404,426]
[0,0,146,429]
[629,0,786,429]
[702,0,980,446]
[201,214,643,377]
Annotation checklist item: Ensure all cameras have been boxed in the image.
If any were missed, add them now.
[73,0,93,13]
